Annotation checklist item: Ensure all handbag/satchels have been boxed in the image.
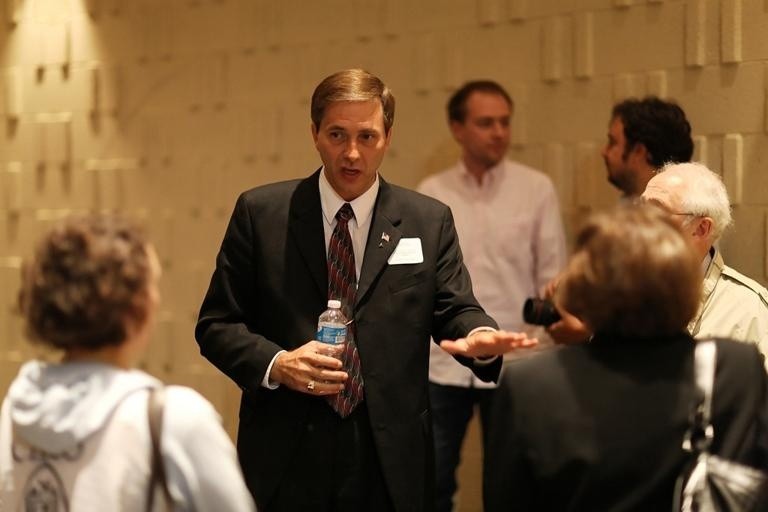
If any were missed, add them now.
[673,340,768,512]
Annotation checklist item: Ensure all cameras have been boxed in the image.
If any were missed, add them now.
[522,297,562,325]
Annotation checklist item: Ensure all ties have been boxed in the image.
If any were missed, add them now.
[325,202,366,419]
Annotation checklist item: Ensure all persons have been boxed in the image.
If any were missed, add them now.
[414,79,567,508]
[195,68,538,512]
[479,206,767,511]
[0,212,258,512]
[541,162,767,369]
[600,94,693,199]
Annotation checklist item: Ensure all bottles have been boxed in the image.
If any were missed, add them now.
[318,299,349,400]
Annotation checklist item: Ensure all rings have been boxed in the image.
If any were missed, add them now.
[307,380,315,393]
